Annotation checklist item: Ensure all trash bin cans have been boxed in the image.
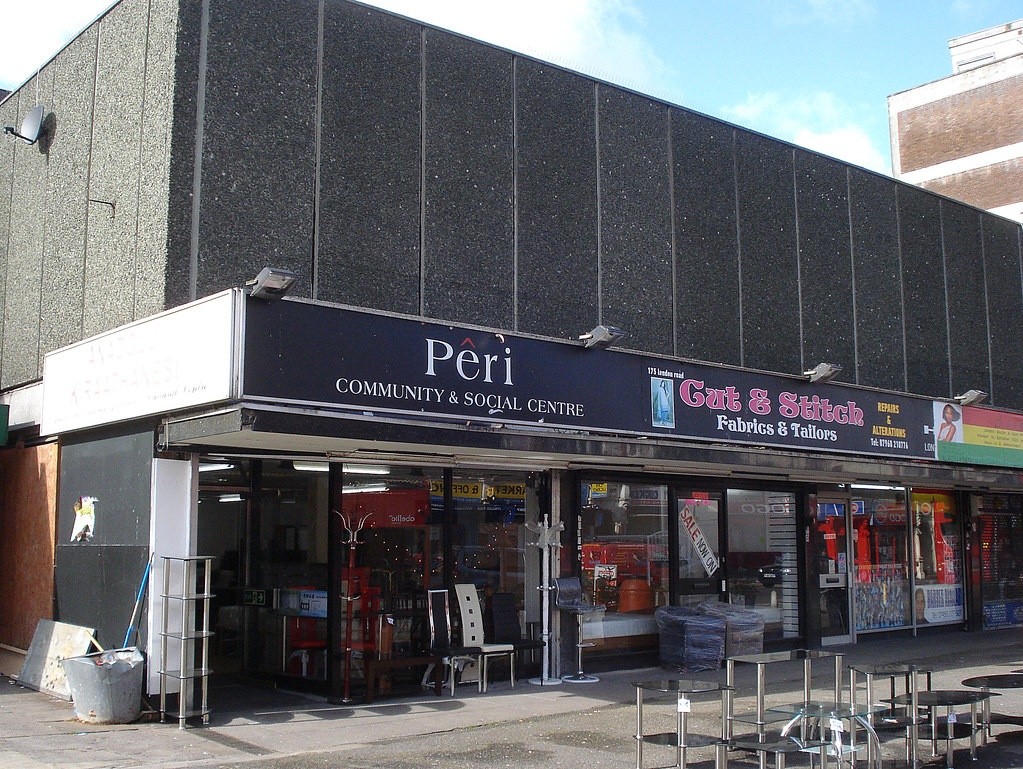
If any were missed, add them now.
[60,647,144,725]
[694,601,766,660]
[655,605,727,673]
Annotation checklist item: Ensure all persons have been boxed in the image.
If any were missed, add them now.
[657,380,670,426]
[915,587,930,625]
[938,404,961,442]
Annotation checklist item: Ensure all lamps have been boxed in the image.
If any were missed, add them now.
[244,266,294,297]
[953,390,989,407]
[579,325,628,348]
[801,361,842,383]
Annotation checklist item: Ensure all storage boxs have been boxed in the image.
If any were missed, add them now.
[656,604,726,673]
[697,604,769,662]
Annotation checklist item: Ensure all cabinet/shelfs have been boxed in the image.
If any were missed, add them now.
[631,649,1003,769]
[157,554,218,731]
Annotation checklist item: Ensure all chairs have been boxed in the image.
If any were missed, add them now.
[488,592,547,686]
[422,589,483,696]
[283,586,327,678]
[455,583,515,693]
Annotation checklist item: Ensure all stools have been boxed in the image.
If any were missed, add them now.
[553,575,606,684]
